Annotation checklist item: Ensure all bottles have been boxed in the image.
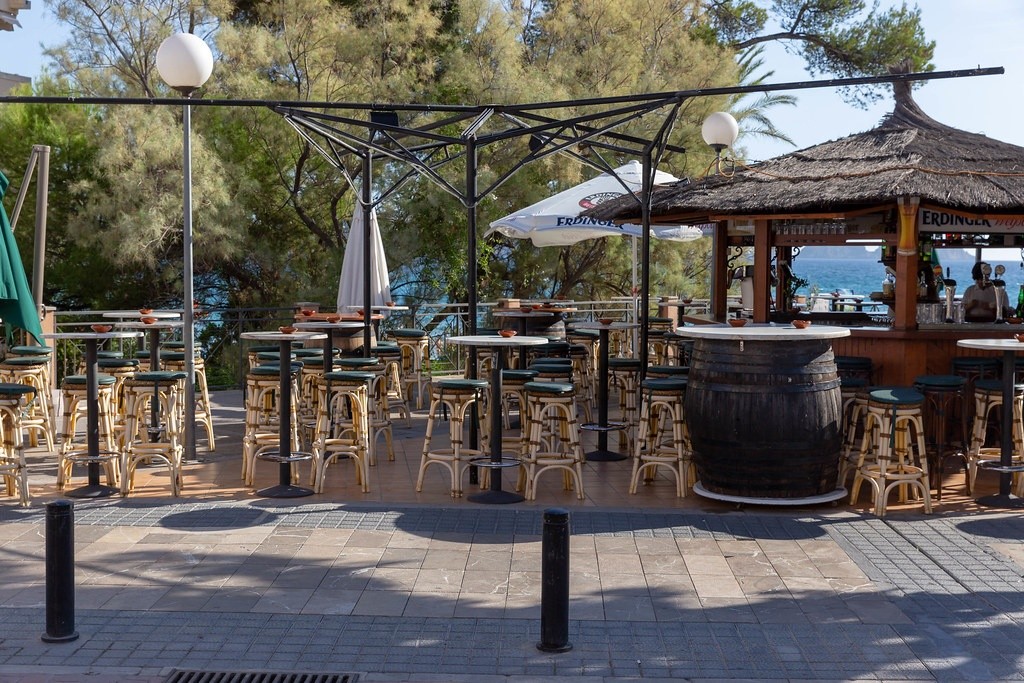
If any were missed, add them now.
[883,274,894,296]
[1017,285,1024,319]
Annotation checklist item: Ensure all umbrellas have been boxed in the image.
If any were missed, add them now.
[481,161,713,360]
[335,180,392,319]
[0,170,45,351]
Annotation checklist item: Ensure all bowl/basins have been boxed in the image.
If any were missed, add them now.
[301,310,315,316]
[543,305,552,308]
[326,317,342,323]
[556,296,566,300]
[279,327,298,334]
[140,318,158,324]
[1014,334,1024,342]
[599,319,613,325]
[532,305,542,309]
[520,308,532,313]
[91,325,112,333]
[738,301,742,304]
[682,300,692,303]
[357,310,365,315]
[384,302,395,306]
[194,303,199,309]
[497,330,517,337]
[138,309,153,314]
[728,319,748,327]
[791,320,811,328]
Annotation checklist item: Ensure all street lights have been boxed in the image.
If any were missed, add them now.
[155,30,215,459]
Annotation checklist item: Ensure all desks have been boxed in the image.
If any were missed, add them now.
[568,322,642,462]
[493,312,554,429]
[657,301,706,367]
[294,313,383,357]
[240,332,327,498]
[346,306,409,341]
[293,322,366,460]
[103,312,180,350]
[957,339,1024,509]
[37,332,145,500]
[446,336,549,505]
[115,321,183,461]
[675,323,851,511]
[492,308,577,339]
[727,295,883,318]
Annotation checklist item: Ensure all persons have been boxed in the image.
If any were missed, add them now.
[960,262,1008,323]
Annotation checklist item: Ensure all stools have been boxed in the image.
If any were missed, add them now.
[0,316,1024,518]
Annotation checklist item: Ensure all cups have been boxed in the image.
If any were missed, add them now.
[916,304,965,324]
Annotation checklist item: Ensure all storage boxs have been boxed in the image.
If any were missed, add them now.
[497,298,520,308]
